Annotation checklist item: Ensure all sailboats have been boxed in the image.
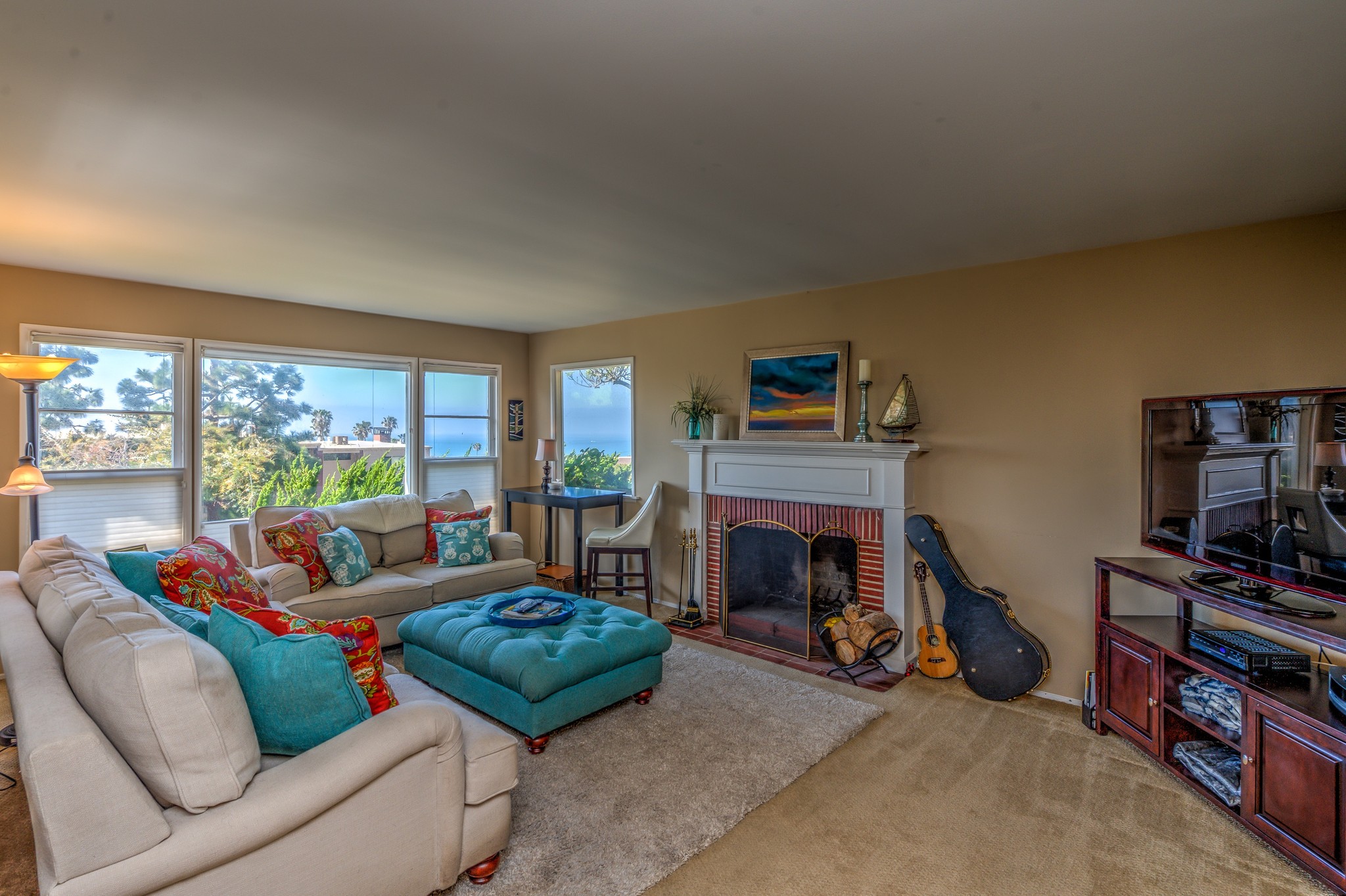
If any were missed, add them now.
[875,373,922,442]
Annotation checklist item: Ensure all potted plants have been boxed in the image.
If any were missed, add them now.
[1257,407,1308,443]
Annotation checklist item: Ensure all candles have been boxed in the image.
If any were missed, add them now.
[859,360,871,382]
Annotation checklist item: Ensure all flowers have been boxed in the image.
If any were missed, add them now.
[670,372,733,445]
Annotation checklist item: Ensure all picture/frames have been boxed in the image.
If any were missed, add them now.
[739,341,849,441]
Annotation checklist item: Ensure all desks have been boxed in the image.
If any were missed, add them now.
[500,485,626,596]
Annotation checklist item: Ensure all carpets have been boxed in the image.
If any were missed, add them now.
[378,641,885,896]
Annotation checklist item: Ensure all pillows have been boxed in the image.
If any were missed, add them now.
[430,517,496,568]
[105,548,183,603]
[420,505,496,565]
[208,604,373,756]
[156,535,272,615]
[261,508,332,593]
[227,599,400,716]
[150,594,209,643]
[317,525,373,587]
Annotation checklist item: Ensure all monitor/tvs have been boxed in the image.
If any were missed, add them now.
[1140,387,1346,619]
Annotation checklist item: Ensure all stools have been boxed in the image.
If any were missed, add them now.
[397,586,672,754]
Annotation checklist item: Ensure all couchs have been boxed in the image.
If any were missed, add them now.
[229,489,537,648]
[0,533,518,896]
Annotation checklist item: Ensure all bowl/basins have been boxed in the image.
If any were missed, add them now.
[548,482,562,489]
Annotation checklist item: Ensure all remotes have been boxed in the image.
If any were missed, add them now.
[517,599,544,613]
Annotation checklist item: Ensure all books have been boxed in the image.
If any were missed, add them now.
[499,598,563,620]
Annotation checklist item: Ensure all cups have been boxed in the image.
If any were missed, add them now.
[1248,417,1271,443]
[713,414,729,440]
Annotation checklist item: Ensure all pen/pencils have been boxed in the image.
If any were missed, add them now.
[547,608,563,616]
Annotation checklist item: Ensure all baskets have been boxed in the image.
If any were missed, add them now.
[535,561,588,593]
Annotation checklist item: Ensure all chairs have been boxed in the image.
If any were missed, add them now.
[585,481,662,618]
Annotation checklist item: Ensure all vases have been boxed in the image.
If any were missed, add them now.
[689,418,700,439]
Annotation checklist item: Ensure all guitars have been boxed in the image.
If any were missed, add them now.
[912,561,961,679]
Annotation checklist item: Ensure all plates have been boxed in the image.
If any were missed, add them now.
[1321,489,1344,495]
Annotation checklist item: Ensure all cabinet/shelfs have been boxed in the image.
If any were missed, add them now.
[1093,556,1346,896]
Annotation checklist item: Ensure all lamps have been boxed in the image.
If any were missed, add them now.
[534,439,559,488]
[0,352,80,545]
[1313,442,1346,495]
